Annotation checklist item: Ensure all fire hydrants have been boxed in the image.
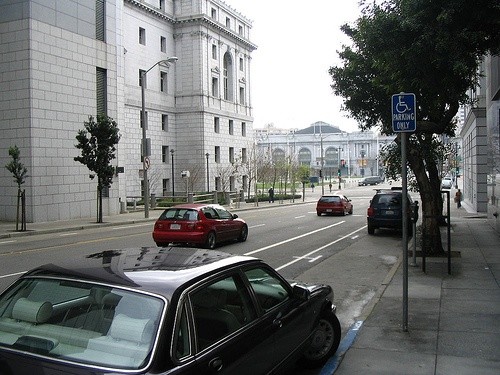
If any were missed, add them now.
[454,189,463,208]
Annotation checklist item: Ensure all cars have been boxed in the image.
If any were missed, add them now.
[152,203,249,248]
[367,189,419,236]
[358,176,380,186]
[316,194,353,216]
[0,247,342,375]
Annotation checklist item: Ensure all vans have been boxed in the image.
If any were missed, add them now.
[441,178,452,188]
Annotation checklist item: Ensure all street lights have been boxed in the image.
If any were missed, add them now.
[452,141,462,189]
[336,146,344,191]
[140,55,179,220]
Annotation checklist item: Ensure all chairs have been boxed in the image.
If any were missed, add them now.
[0,291,153,360]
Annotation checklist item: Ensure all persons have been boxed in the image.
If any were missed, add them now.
[267,187,275,204]
[455,189,463,208]
